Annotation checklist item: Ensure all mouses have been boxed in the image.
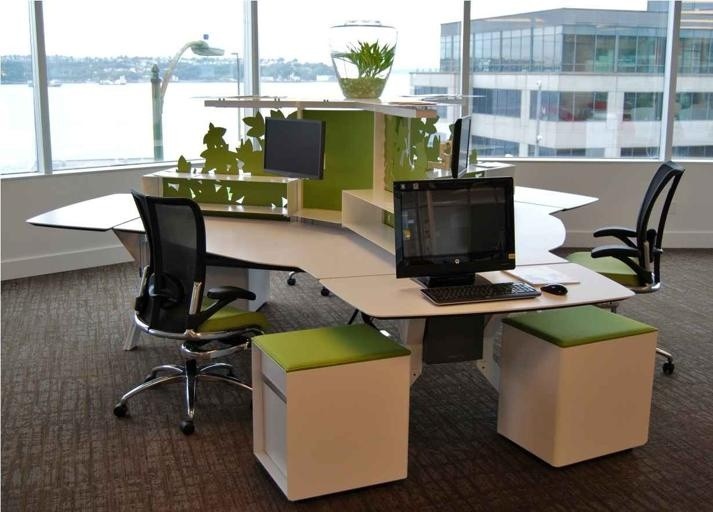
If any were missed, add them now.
[545,283,568,294]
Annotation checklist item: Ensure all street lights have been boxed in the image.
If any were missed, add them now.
[138,41,225,162]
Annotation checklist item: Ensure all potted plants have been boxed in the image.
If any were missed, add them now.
[329,26,396,98]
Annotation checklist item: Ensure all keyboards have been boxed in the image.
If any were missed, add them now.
[420,282,539,306]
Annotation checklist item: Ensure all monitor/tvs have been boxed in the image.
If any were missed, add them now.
[263,116,327,183]
[449,114,472,176]
[392,176,517,286]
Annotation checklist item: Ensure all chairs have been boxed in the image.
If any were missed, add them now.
[564,161,687,373]
[113,189,265,435]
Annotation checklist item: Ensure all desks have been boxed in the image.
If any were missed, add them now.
[26,185,635,387]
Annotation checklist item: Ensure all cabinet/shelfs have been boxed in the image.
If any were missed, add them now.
[494,304,659,469]
[141,94,485,256]
[249,322,412,501]
[202,262,270,314]
